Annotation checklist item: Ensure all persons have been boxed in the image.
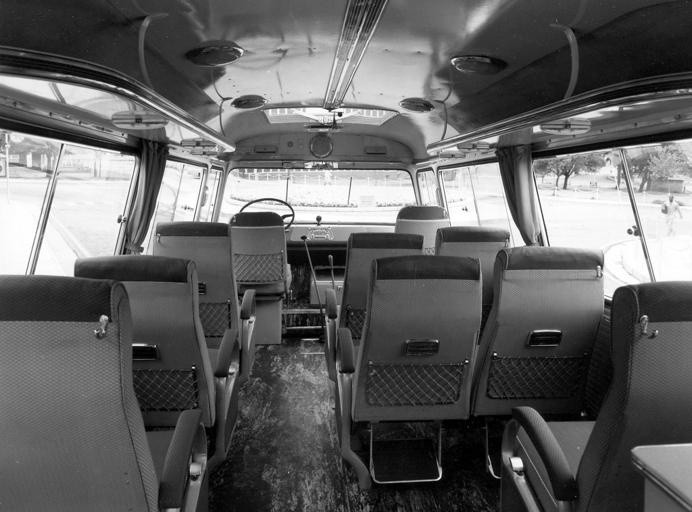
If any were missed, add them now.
[661,194,683,236]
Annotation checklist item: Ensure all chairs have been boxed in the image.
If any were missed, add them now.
[72,252,239,474]
[228,211,291,304]
[472,245,607,484]
[393,204,453,256]
[150,219,257,380]
[2,272,213,511]
[324,231,425,384]
[485,274,691,511]
[334,256,485,489]
[433,225,512,345]
